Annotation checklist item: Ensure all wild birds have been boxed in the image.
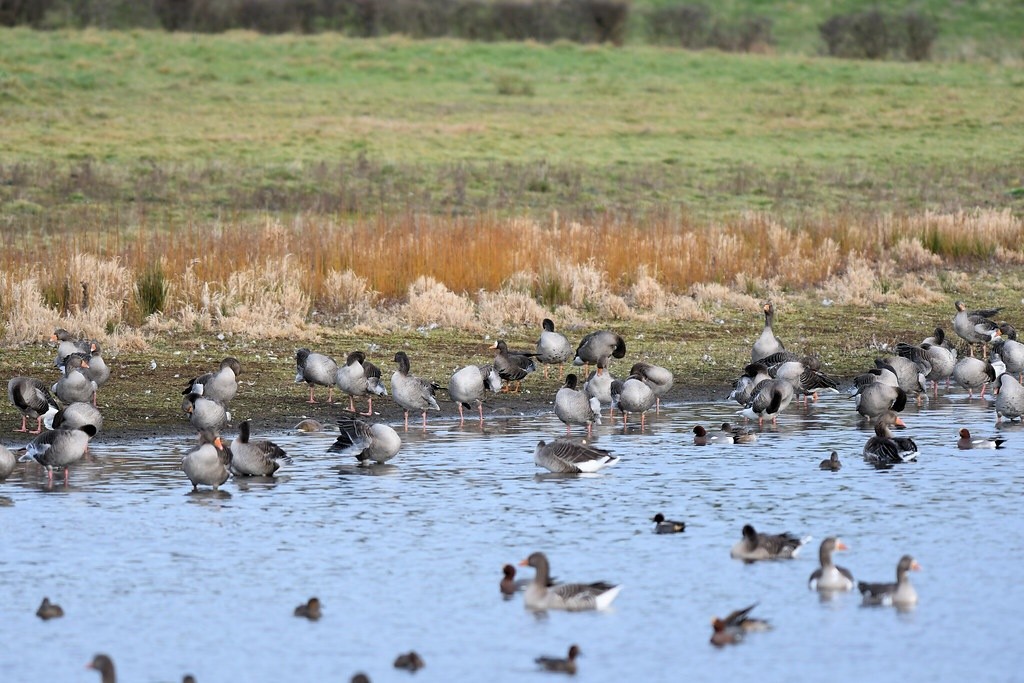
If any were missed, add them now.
[691,423,758,446]
[0,328,110,489]
[536,319,674,437]
[37,598,64,617]
[181,358,289,492]
[855,555,922,610]
[534,440,620,473]
[288,415,402,467]
[395,652,424,672]
[728,302,842,427]
[819,450,842,470]
[87,653,116,683]
[391,351,448,432]
[650,513,685,533]
[729,523,808,560]
[534,645,585,674]
[447,365,502,428]
[294,598,323,620]
[711,603,775,645]
[295,348,388,415]
[847,299,1024,465]
[501,552,623,611]
[489,339,544,394]
[808,537,856,590]
